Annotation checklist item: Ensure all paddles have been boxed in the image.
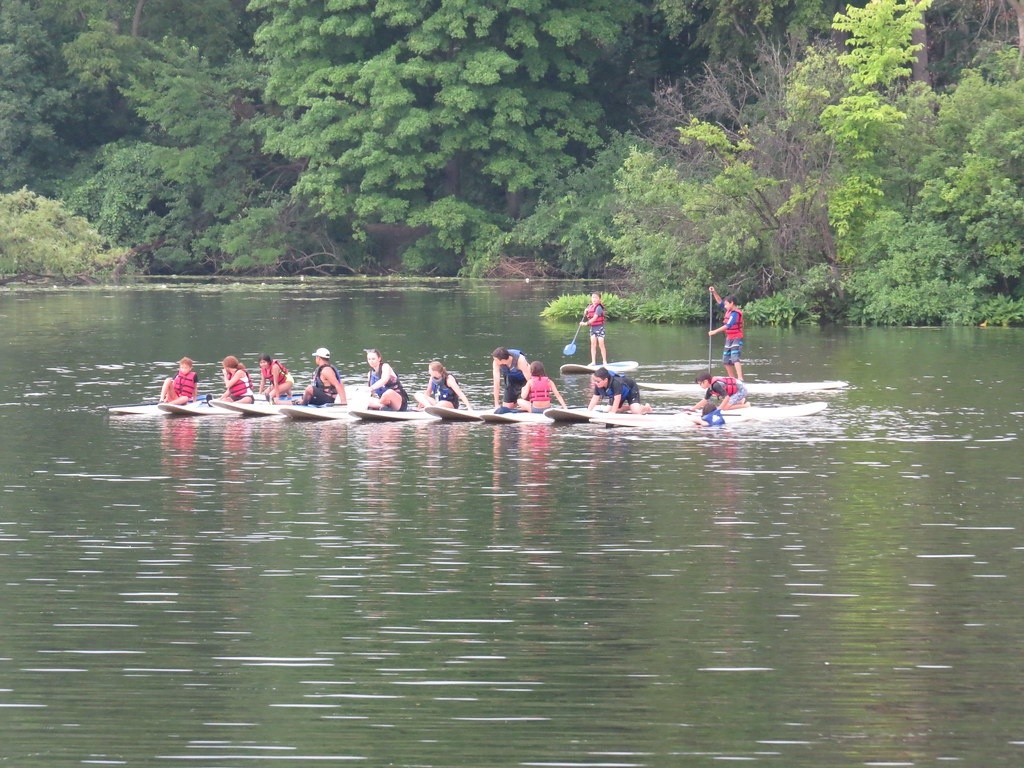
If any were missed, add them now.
[563,312,586,355]
[705,289,715,378]
[643,411,743,418]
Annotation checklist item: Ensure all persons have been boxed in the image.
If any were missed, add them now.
[293,348,347,407]
[579,291,607,367]
[708,287,744,384]
[517,361,567,414]
[258,353,294,405]
[158,357,198,406]
[693,403,726,426]
[491,348,531,412]
[587,367,653,416]
[218,356,255,405]
[690,373,751,412]
[363,349,408,412]
[417,362,472,412]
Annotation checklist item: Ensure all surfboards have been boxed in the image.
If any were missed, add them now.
[215,402,288,416]
[425,406,497,420]
[480,412,557,424]
[560,360,641,375]
[157,403,242,415]
[543,409,608,420]
[635,380,849,396]
[108,403,171,416]
[279,403,360,420]
[591,401,828,428]
[349,408,439,420]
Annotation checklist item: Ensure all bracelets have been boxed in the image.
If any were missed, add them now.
[587,322,591,327]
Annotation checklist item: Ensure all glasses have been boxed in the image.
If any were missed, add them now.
[594,379,604,383]
[367,349,378,354]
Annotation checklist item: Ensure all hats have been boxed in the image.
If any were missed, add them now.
[312,348,330,358]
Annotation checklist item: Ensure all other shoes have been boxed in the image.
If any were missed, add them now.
[381,405,392,412]
[206,393,213,407]
